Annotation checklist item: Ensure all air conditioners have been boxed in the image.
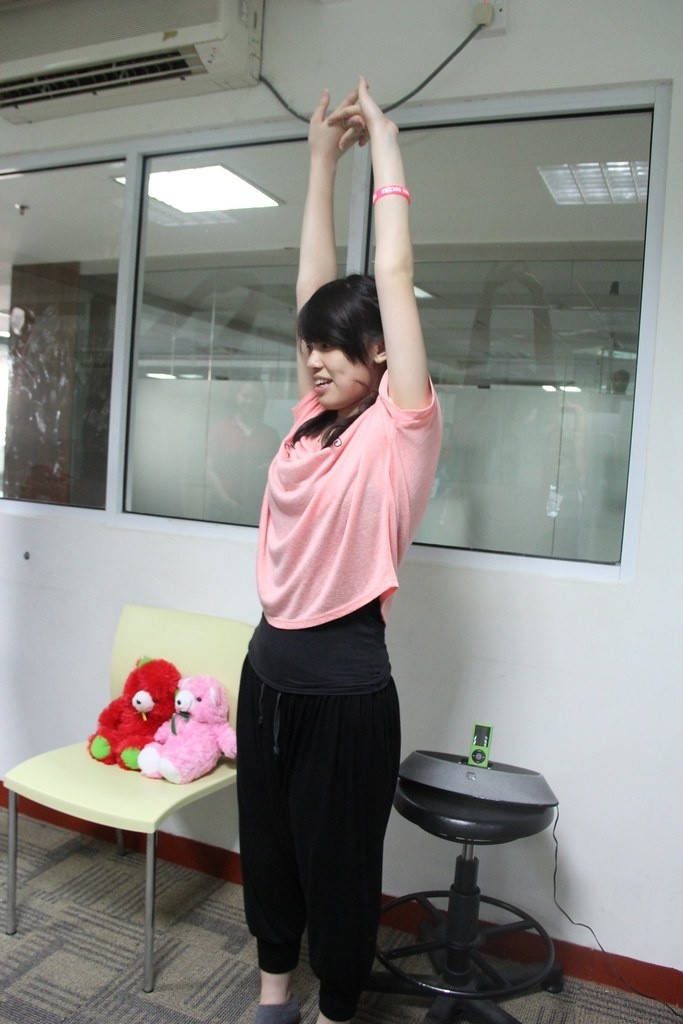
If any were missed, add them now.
[0,0,266,124]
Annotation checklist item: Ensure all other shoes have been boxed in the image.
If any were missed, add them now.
[251,992,300,1024]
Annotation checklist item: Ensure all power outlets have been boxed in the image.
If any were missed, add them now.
[469,0,510,39]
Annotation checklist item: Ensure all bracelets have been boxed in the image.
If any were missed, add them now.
[372,184,411,204]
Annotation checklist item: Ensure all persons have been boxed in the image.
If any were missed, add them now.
[236,75,444,1024]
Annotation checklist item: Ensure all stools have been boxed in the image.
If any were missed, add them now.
[370,778,567,1024]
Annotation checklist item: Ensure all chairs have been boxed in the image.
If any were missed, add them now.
[4,603,257,993]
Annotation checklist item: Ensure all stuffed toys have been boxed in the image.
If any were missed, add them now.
[90,653,236,784]
